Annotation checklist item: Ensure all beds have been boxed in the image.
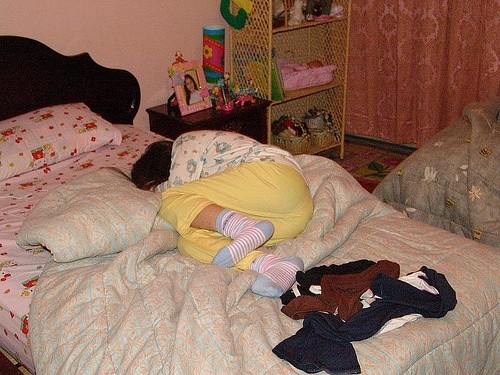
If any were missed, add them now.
[0,35,500,375]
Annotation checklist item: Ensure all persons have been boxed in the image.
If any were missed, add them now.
[183,74,204,107]
[132,130,314,299]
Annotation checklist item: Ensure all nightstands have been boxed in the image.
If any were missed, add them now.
[146,97,273,144]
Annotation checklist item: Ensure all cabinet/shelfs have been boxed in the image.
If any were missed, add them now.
[231,0,353,161]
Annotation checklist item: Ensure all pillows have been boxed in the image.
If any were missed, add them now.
[0,103,122,179]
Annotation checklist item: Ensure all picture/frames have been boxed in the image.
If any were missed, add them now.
[169,60,213,116]
